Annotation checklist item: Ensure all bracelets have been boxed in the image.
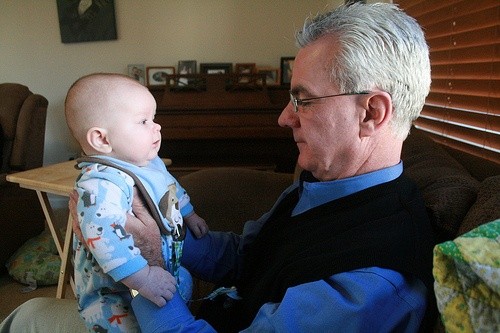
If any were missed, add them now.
[129,288,139,299]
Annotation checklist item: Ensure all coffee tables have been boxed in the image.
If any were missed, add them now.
[7,157,172,300]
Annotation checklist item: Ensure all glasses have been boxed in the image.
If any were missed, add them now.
[289,90,370,113]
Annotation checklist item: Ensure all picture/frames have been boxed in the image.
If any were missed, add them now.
[127,56,295,90]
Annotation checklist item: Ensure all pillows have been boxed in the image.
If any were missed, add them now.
[9,219,67,288]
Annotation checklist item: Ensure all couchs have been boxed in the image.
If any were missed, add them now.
[177,129,500,332]
[0,83,49,277]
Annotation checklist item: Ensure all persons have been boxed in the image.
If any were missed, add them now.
[0,1,438,333]
[64,72,208,333]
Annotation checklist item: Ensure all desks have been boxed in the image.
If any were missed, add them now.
[145,84,296,140]
